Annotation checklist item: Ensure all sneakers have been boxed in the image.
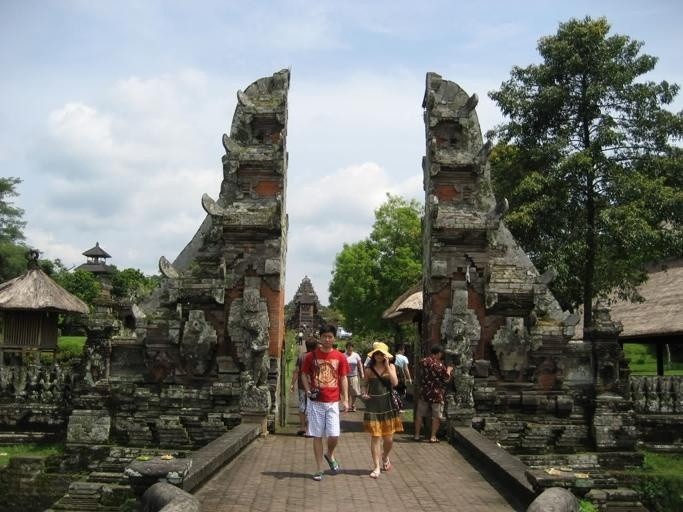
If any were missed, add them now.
[352,404,356,411]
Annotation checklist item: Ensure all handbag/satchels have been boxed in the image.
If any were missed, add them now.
[390,389,402,412]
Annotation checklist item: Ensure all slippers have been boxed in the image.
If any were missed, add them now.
[369,469,380,479]
[381,457,390,470]
[313,470,325,480]
[297,431,304,435]
[429,439,439,443]
[324,454,339,473]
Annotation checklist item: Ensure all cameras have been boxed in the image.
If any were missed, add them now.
[310,390,320,401]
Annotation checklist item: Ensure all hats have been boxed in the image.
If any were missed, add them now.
[367,342,393,359]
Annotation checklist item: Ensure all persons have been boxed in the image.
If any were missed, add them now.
[299,324,352,482]
[342,342,365,412]
[298,329,303,344]
[360,342,405,478]
[388,353,408,402]
[393,344,413,386]
[362,342,381,375]
[412,344,454,443]
[288,336,319,437]
